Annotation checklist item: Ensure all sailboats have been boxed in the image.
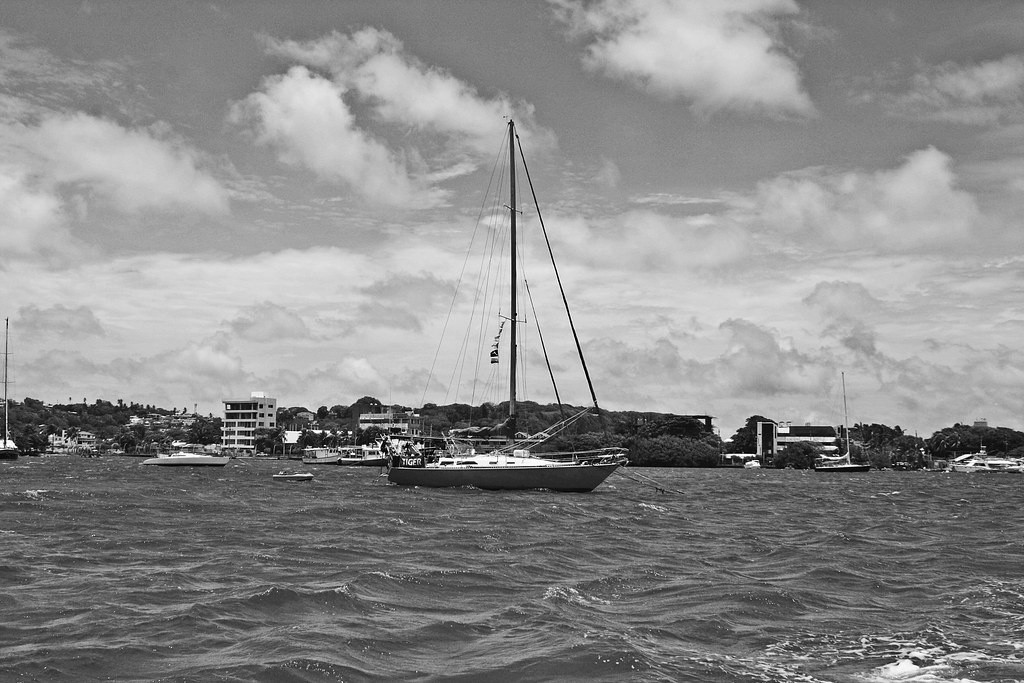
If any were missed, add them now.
[947,435,1024,473]
[812,369,872,472]
[300,113,632,493]
[0,316,20,458]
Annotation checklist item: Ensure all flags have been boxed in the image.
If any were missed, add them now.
[490,321,505,364]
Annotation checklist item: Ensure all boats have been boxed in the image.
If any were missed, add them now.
[742,459,761,470]
[272,472,315,481]
[142,451,232,465]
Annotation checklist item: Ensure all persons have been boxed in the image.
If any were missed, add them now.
[380,437,391,457]
